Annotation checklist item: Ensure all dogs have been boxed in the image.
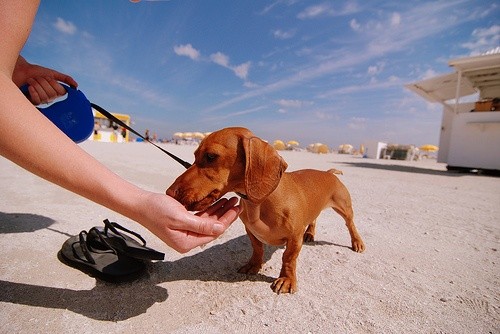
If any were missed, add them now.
[166,127,365,294]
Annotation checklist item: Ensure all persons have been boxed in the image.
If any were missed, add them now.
[0,0,244,255]
[490,97,500,111]
[95,119,171,143]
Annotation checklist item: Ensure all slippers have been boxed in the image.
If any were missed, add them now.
[89,219,166,262]
[59,231,142,285]
